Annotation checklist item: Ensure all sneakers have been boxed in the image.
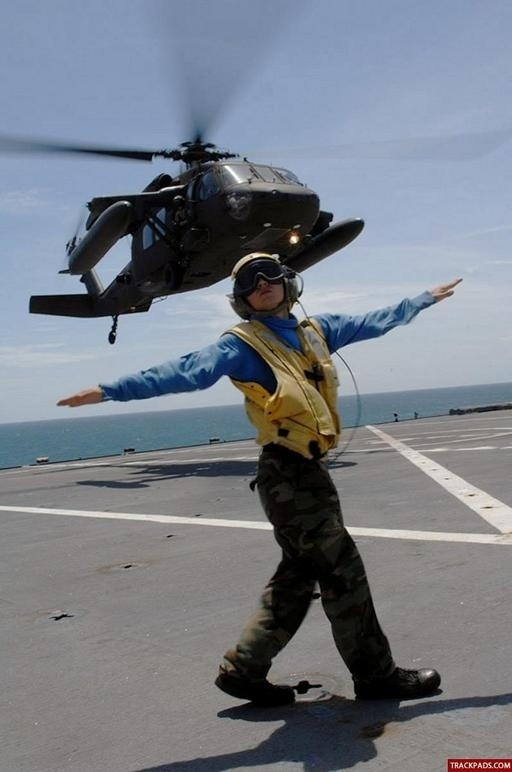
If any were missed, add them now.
[215,675,294,703]
[353,667,440,698]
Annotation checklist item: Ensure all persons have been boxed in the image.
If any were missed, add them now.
[54,249,463,709]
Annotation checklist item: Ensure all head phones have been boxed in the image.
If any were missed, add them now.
[230,276,299,319]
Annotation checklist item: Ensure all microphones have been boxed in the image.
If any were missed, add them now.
[281,264,304,298]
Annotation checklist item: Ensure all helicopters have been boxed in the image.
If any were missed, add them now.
[2,131,364,344]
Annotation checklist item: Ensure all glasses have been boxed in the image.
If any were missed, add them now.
[236,260,283,296]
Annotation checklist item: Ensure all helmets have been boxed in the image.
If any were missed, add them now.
[226,252,303,317]
